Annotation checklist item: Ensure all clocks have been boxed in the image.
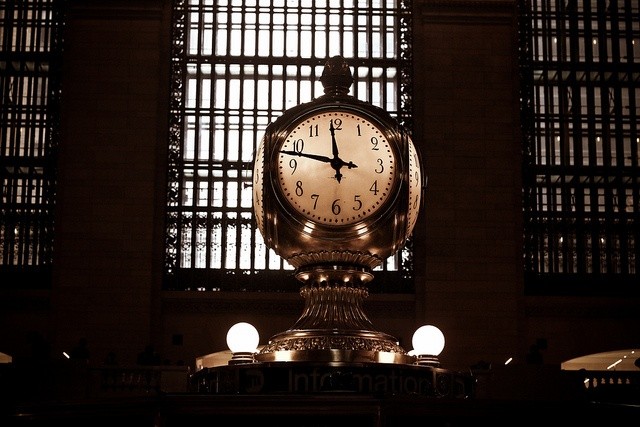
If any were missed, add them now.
[254,55,423,362]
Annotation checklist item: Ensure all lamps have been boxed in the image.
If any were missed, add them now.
[225,321,260,366]
[412,324,446,366]
